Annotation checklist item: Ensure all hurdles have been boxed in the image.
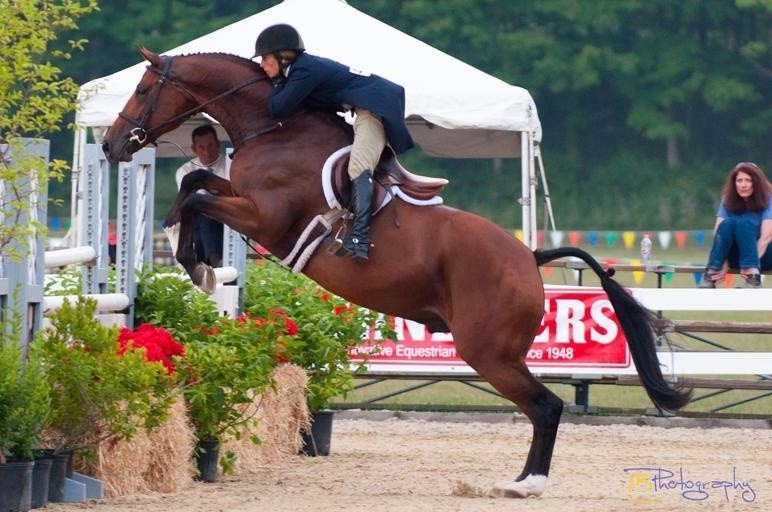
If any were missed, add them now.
[0,137,248,367]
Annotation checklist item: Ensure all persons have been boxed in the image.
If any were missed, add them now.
[251,24,416,262]
[697,161,772,288]
[175,124,225,268]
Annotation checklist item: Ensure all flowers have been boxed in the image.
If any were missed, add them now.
[134,261,299,474]
[248,262,398,410]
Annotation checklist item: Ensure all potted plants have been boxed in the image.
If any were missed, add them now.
[0,292,169,512]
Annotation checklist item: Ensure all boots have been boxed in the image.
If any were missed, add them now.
[324,169,375,263]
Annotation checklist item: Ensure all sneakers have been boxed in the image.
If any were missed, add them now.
[698,271,716,288]
[744,273,761,288]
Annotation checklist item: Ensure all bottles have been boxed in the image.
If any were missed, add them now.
[640,234,653,268]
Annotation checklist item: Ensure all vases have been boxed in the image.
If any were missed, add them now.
[298,412,332,456]
[195,438,219,483]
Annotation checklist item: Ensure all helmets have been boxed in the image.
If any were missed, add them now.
[249,23,305,61]
